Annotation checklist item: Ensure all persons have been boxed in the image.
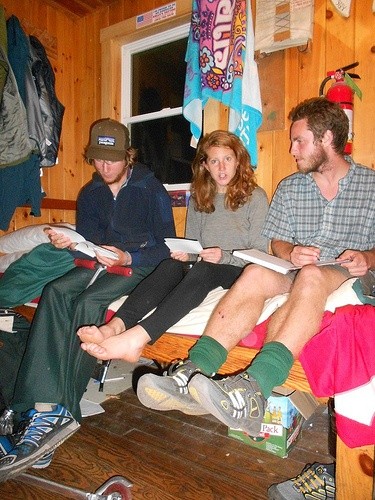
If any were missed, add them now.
[75,131,266,364]
[137,98,375,435]
[0,118,177,479]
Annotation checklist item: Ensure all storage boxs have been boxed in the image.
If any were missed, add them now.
[228,387,320,458]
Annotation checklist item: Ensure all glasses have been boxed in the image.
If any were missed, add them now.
[91,158,121,165]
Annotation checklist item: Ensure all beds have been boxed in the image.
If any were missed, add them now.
[0,273,375,500]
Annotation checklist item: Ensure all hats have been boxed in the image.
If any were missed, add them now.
[85,118,130,161]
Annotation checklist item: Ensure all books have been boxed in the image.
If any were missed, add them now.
[232,248,350,275]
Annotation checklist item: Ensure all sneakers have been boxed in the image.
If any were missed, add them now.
[265,462,335,500]
[136,361,211,416]
[188,370,268,438]
[0,410,54,469]
[0,403,81,483]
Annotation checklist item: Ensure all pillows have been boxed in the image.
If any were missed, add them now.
[0,222,76,253]
[0,251,30,273]
